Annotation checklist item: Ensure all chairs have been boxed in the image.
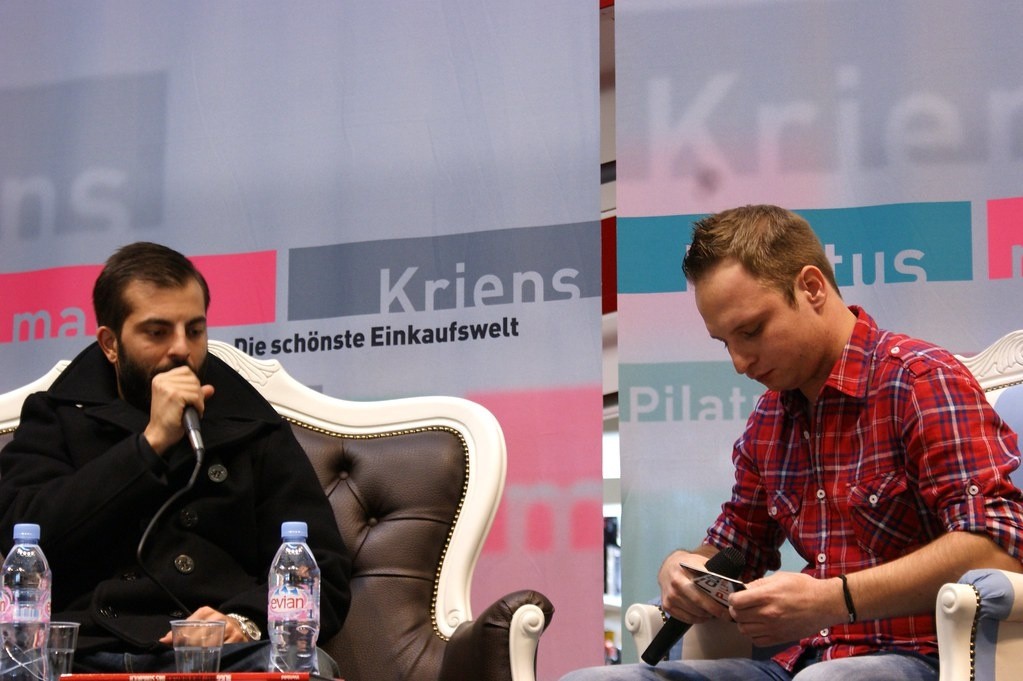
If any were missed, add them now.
[0,338,557,681]
[623,322,1023,681]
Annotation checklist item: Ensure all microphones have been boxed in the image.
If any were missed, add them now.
[641,548,747,667]
[181,405,206,464]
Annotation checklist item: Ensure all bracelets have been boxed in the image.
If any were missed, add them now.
[837,574,857,623]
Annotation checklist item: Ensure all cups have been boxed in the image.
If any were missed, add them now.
[169,620,227,673]
[48,621,80,681]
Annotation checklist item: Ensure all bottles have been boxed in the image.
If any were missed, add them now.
[0,523,53,681]
[267,521,322,676]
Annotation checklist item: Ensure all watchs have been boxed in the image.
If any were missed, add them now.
[225,613,261,642]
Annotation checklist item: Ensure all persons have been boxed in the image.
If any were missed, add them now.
[0,241,353,678]
[556,204,1023,681]
